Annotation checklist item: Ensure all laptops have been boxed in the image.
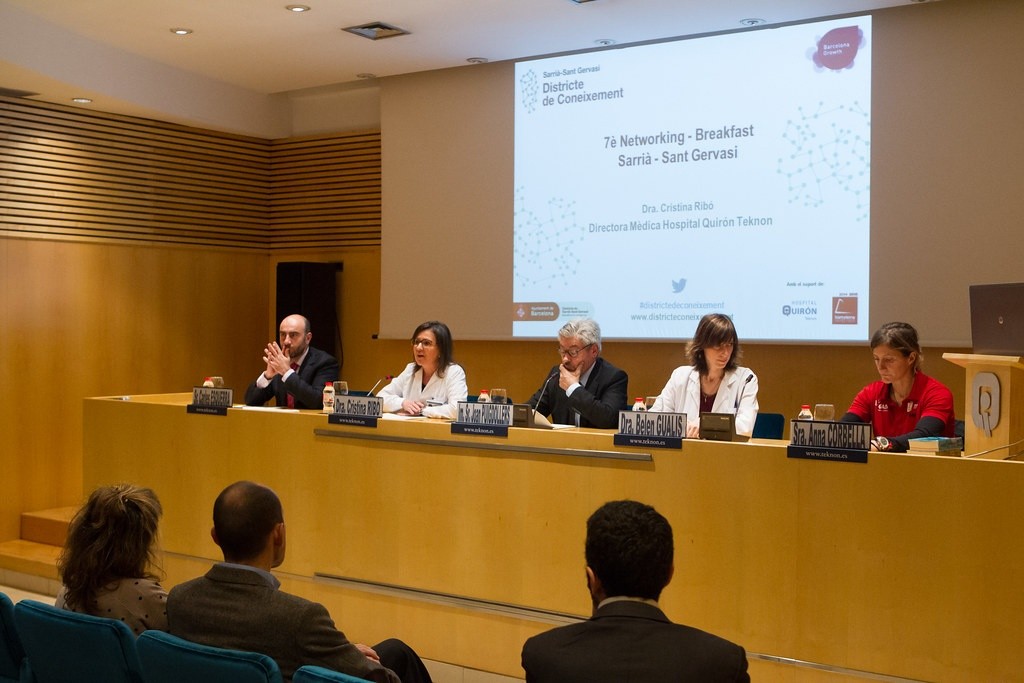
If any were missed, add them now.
[969,282,1024,357]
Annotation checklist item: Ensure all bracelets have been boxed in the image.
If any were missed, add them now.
[870,440,881,450]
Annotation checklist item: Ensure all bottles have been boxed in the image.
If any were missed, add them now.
[322,382,335,414]
[203,377,214,388]
[798,405,813,420]
[477,390,490,403]
[632,398,647,412]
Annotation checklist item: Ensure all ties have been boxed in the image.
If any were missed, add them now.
[287,363,299,409]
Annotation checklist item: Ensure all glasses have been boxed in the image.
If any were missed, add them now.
[555,343,591,358]
[411,339,438,347]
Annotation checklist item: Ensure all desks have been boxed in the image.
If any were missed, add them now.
[82,391,1024,683]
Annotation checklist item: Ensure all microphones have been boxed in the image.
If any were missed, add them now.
[367,374,394,396]
[533,372,559,422]
[733,374,753,441]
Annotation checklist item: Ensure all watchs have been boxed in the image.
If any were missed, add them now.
[874,436,889,452]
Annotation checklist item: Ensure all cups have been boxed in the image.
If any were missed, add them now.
[211,376,224,388]
[489,389,507,404]
[813,403,835,422]
[333,381,348,396]
[645,396,663,413]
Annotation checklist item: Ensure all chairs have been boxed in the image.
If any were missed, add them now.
[14,599,143,683]
[751,412,784,440]
[133,631,281,683]
[0,592,14,683]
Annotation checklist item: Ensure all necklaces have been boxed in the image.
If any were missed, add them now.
[702,371,723,402]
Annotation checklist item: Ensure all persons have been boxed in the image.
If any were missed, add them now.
[372,320,468,418]
[645,314,759,438]
[836,322,956,455]
[54,485,170,638]
[520,498,755,683]
[166,478,435,683]
[518,316,629,428]
[244,314,339,408]
